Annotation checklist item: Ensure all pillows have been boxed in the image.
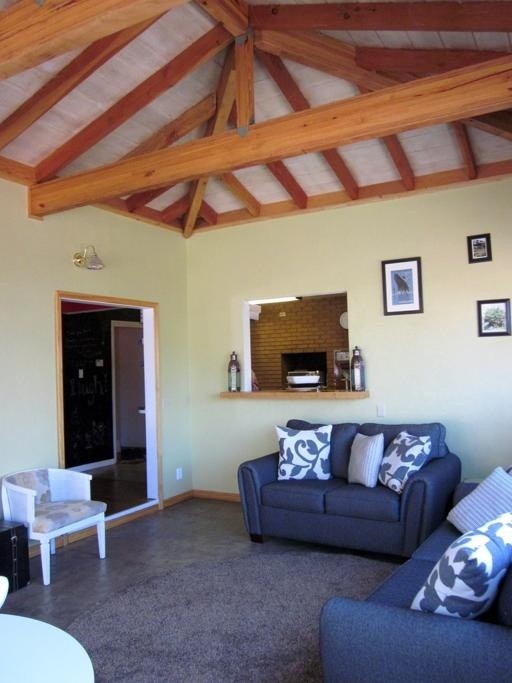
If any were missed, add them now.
[411,512,512,620]
[274,425,333,481]
[348,432,384,488]
[447,465,512,534]
[379,431,432,494]
[358,422,446,483]
[286,420,360,478]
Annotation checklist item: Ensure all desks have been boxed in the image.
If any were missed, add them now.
[0,613,95,683]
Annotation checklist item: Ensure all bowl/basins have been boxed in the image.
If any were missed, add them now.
[286,375,321,392]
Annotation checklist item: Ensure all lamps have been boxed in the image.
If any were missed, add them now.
[74,244,105,271]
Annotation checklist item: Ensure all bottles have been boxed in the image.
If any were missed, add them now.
[227,350,240,392]
[350,345,366,392]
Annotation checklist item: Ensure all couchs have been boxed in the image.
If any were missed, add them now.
[319,468,512,683]
[237,419,461,564]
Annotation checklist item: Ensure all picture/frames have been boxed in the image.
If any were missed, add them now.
[477,299,512,337]
[381,256,423,316]
[466,233,492,264]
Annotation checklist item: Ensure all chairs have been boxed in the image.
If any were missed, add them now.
[2,467,108,586]
